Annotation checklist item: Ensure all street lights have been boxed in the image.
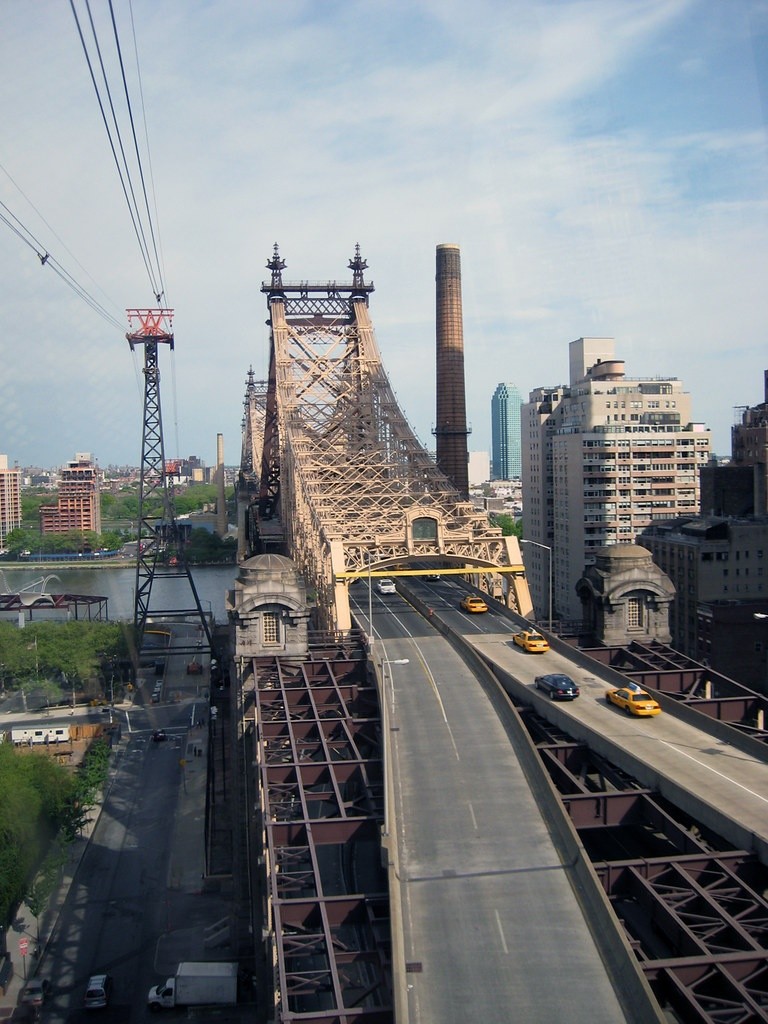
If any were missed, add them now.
[520,539,552,632]
[382,658,409,711]
[369,554,386,644]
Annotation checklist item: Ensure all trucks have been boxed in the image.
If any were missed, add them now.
[147,962,238,1013]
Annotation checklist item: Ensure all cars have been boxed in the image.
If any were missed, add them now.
[152,680,162,703]
[606,682,661,716]
[394,564,411,571]
[84,975,111,1011]
[535,674,579,700]
[20,978,51,1006]
[377,579,396,595]
[420,575,440,582]
[513,627,550,653]
[460,593,488,614]
[153,729,165,741]
[11,1006,42,1024]
